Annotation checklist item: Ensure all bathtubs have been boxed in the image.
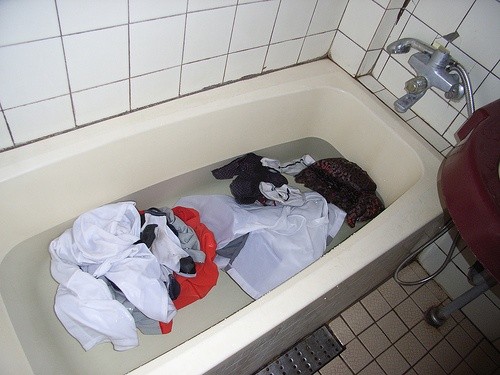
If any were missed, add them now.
[0,52,459,374]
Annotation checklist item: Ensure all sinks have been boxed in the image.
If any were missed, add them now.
[441,95,500,287]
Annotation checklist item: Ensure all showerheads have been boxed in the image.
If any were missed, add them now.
[386,36,456,67]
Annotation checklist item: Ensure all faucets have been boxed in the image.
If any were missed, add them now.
[392,82,433,114]
